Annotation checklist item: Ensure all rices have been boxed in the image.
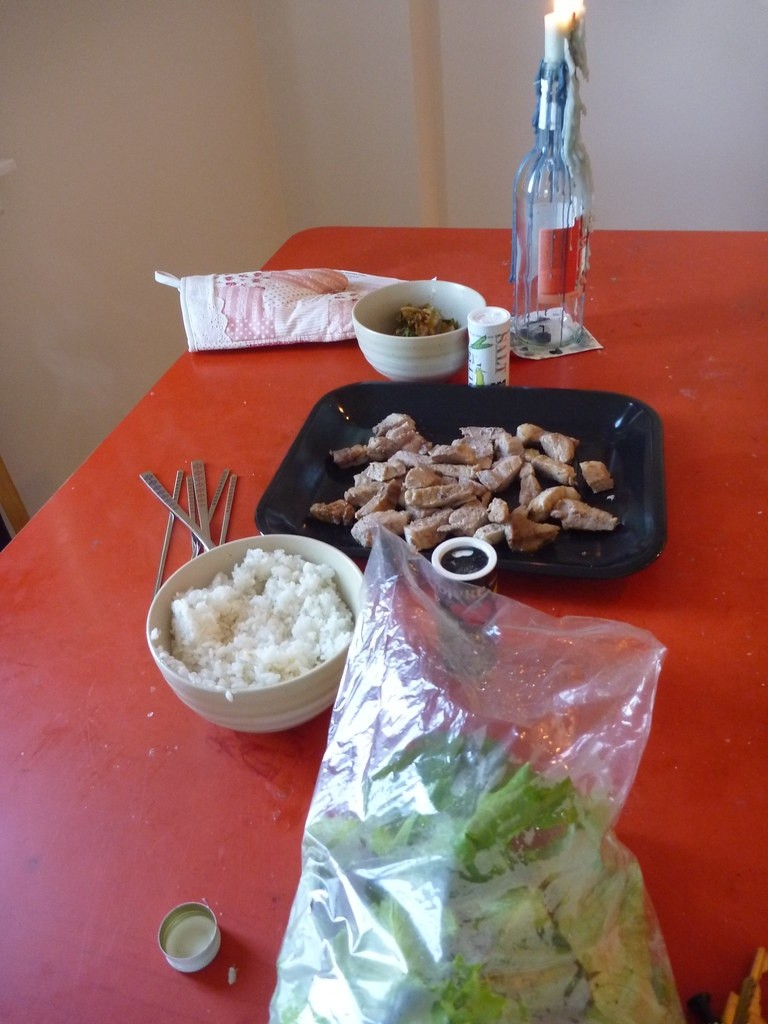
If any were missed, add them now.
[153,546,354,703]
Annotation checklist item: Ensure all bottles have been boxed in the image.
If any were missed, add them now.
[515,59,591,348]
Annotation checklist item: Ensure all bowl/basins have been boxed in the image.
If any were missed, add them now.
[145,534,366,732]
[352,280,485,380]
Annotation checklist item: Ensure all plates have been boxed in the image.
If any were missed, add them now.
[256,380,667,580]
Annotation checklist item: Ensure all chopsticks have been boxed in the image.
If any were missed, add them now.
[139,459,237,601]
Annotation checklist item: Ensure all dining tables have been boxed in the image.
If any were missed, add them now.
[0,228,768,1024]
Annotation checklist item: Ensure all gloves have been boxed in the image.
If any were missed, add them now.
[155,268,436,353]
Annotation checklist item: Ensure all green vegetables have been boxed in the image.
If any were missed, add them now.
[262,725,687,1024]
